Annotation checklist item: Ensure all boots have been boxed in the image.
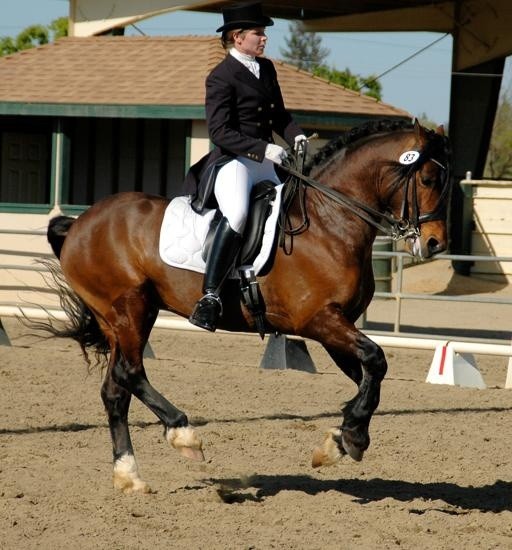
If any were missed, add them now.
[189,216,241,332]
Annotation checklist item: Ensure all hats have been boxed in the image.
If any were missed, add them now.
[216,4,274,32]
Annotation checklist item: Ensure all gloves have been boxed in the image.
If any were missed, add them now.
[294,134,308,155]
[264,143,288,165]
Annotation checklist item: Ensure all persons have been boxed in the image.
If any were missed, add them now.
[187,7,309,331]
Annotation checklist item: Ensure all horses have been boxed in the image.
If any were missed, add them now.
[11,118,455,495]
